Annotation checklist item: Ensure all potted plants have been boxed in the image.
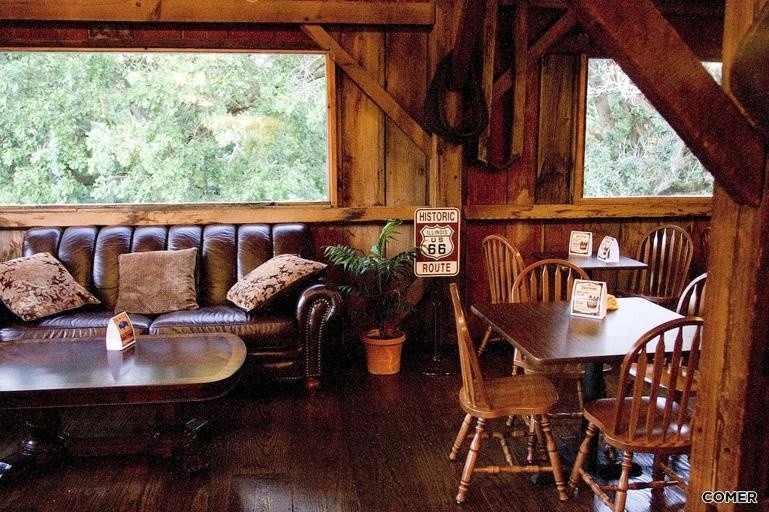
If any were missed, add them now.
[325,219,427,375]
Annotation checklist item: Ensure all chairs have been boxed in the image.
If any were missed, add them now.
[619,223,693,313]
[511,258,621,463]
[626,271,709,461]
[447,280,571,507]
[568,312,708,512]
[700,224,710,267]
[476,233,551,377]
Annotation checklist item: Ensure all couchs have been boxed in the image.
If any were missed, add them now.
[0,222,343,395]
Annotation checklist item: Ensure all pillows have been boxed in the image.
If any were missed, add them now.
[226,253,329,313]
[0,253,100,319]
[113,246,201,317]
